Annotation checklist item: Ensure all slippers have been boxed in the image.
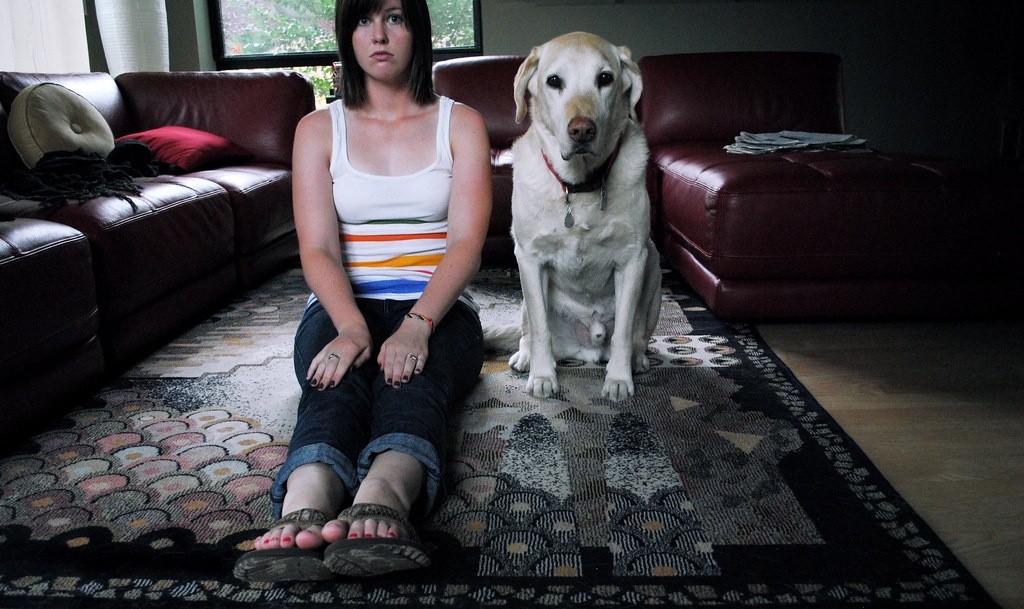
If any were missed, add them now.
[233,509,329,581]
[323,503,434,573]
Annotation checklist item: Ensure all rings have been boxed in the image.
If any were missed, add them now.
[406,355,417,362]
[329,354,340,361]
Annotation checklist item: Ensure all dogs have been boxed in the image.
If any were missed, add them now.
[482,32,660,404]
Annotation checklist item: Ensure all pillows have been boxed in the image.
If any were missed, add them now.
[116,125,234,170]
[7,81,116,169]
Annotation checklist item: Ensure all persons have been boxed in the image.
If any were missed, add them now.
[233,0,493,578]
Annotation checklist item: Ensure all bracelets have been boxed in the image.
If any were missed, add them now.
[404,313,434,335]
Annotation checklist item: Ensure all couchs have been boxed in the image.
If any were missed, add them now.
[432,50,1023,321]
[0,73,314,441]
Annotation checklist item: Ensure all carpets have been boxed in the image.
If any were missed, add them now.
[0,238,1001,608]
